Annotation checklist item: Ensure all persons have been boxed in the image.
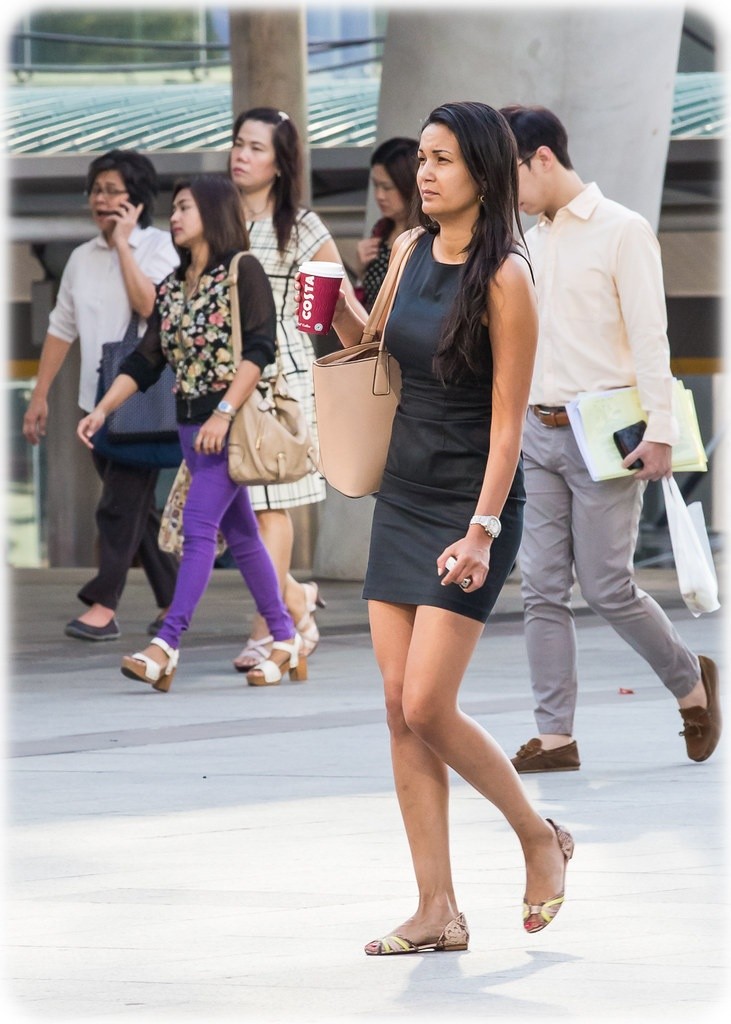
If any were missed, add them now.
[489,102,723,772]
[75,178,307,692]
[294,102,573,957]
[357,137,421,316]
[200,108,371,673]
[24,148,181,643]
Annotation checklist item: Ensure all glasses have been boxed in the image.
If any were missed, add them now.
[84,186,130,198]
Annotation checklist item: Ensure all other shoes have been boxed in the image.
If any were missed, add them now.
[679,656,722,762]
[66,620,122,641]
[510,738,580,773]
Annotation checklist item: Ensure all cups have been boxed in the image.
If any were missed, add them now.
[294,260,344,335]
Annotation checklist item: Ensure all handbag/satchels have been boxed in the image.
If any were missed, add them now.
[661,475,721,618]
[227,397,319,484]
[314,343,401,497]
[95,311,183,470]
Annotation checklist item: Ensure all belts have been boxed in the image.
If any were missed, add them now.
[530,405,569,427]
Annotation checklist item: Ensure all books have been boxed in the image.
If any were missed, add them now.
[565,378,707,482]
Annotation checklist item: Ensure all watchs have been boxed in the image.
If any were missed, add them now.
[469,514,502,539]
[217,401,235,416]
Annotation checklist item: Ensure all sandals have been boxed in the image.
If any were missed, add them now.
[365,912,469,955]
[523,818,574,932]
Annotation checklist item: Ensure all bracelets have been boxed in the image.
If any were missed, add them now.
[213,409,231,421]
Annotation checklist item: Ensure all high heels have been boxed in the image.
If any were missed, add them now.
[248,636,307,683]
[234,634,276,672]
[121,637,179,693]
[294,583,326,658]
